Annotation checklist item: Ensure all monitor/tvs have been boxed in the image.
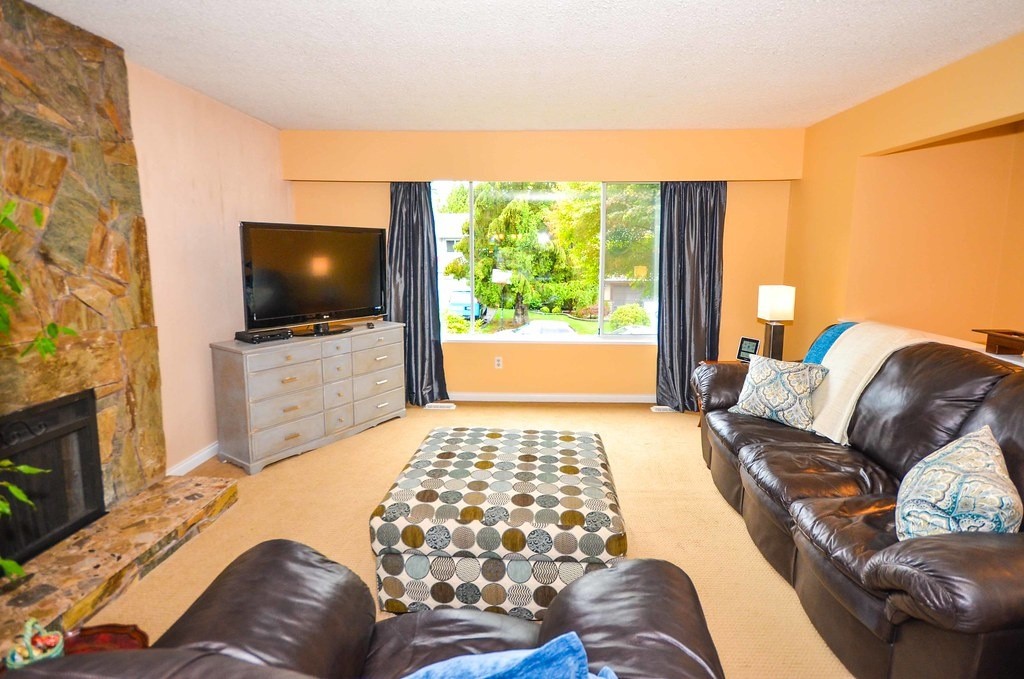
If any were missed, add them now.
[238,220,388,335]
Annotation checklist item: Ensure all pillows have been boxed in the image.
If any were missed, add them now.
[894,424,1023,543]
[728,353,830,431]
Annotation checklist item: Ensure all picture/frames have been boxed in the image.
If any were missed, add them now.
[736,336,760,363]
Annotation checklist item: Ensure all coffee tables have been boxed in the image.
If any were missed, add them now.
[369,427,627,623]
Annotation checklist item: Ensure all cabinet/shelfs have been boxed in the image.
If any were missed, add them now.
[209,321,407,475]
[971,327,1024,356]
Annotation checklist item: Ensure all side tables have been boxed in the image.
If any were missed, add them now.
[697,360,740,428]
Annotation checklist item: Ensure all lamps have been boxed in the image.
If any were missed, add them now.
[757,284,796,362]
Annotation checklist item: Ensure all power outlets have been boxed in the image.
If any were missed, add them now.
[495,357,503,368]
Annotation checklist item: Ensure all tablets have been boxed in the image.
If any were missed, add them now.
[736,336,761,363]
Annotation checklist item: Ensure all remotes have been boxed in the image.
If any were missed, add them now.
[367,323,374,329]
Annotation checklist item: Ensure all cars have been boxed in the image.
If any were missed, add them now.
[448,289,488,320]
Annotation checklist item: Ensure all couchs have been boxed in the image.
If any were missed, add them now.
[2,538,725,679]
[688,321,1024,679]
